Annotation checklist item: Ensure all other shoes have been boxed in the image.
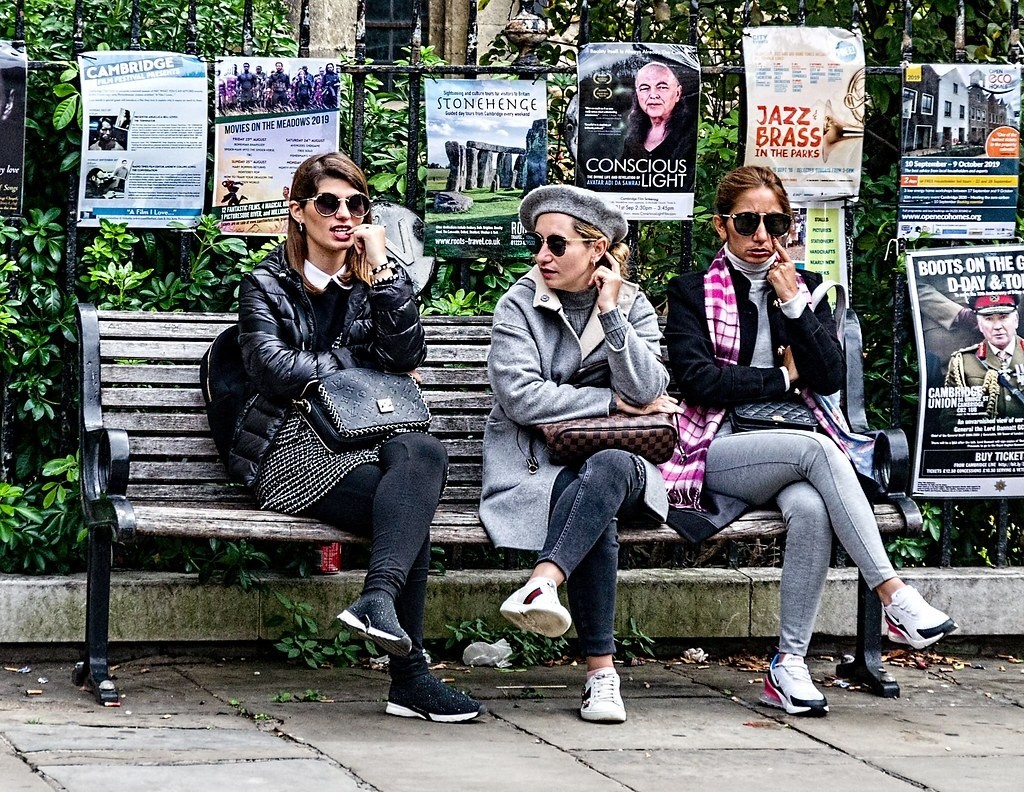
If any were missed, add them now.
[337,596,412,657]
[385,661,487,723]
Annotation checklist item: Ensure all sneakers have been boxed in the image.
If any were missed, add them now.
[759,653,829,715]
[883,585,959,650]
[580,669,627,721]
[500,578,572,638]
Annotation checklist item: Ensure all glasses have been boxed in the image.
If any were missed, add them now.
[719,212,791,237]
[521,231,598,258]
[298,192,371,219]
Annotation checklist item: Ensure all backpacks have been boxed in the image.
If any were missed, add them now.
[200,323,239,465]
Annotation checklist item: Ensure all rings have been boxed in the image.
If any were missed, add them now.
[774,261,778,267]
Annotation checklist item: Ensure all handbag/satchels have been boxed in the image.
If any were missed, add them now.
[729,402,823,433]
[294,367,433,453]
[534,360,678,467]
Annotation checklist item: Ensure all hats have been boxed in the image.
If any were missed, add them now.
[518,184,630,246]
[968,283,1020,316]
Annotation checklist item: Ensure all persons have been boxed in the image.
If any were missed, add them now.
[283,187,289,198]
[665,166,960,719]
[228,153,489,722]
[214,62,341,116]
[478,183,685,722]
[784,213,807,249]
[915,256,1024,439]
[0,75,24,194]
[85,159,129,199]
[577,61,699,192]
[94,109,131,150]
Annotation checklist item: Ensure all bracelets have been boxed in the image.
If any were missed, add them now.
[370,262,396,275]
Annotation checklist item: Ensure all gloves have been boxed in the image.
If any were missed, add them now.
[972,324,983,336]
[960,308,978,330]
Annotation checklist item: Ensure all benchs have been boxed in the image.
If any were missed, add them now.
[65,302,929,715]
